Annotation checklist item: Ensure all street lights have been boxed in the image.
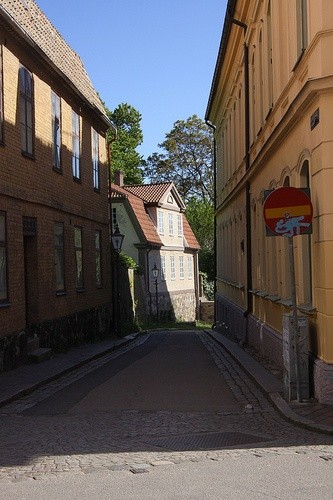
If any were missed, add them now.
[111,225,125,336]
[152,262,161,329]
